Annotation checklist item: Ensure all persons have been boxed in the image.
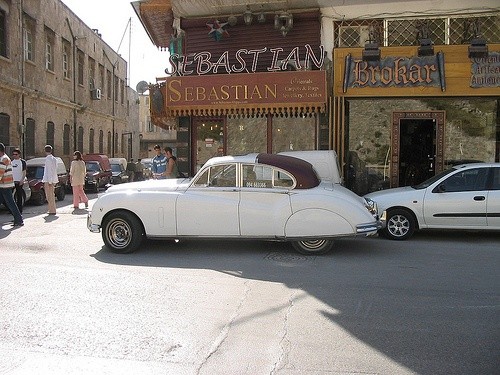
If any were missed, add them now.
[128,158,140,180]
[161,147,177,178]
[9,149,27,215]
[68,151,88,214]
[42,145,58,214]
[151,144,167,179]
[0,143,24,226]
[136,159,143,180]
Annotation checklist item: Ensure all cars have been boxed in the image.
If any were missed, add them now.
[141,158,154,171]
[69,152,113,192]
[24,156,69,206]
[363,163,500,240]
[108,157,128,184]
[87,152,388,255]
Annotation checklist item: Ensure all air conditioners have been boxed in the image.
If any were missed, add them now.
[92,89,102,100]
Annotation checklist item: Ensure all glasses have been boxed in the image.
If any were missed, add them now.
[13,154,19,155]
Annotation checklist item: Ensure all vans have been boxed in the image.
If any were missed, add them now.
[213,149,346,188]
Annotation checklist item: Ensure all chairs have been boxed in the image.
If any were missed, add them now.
[247,169,256,187]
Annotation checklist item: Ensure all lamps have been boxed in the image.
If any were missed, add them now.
[417,38,434,57]
[468,38,489,58]
[362,43,381,62]
[242,4,253,26]
